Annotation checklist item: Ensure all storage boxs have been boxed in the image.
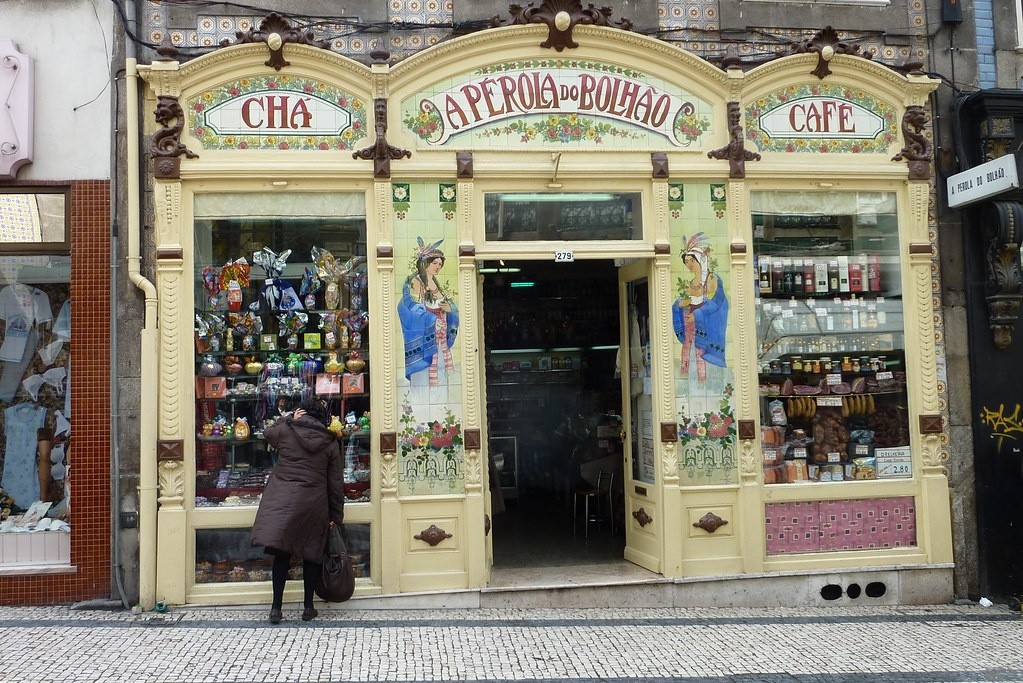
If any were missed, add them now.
[259,333,277,350]
[303,333,321,349]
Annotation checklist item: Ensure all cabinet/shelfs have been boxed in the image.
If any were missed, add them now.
[195,306,370,499]
[487,369,581,475]
[757,248,906,466]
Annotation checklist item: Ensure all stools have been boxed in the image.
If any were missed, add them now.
[573,470,615,539]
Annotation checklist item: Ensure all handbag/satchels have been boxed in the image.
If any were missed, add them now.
[314,523,355,603]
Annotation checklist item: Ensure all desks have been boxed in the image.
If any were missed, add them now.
[553,431,623,535]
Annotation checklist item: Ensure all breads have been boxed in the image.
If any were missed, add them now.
[762,375,908,484]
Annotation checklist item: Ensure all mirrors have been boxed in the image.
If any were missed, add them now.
[489,436,517,489]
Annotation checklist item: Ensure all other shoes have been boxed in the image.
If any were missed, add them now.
[269,608,282,624]
[302,605,318,621]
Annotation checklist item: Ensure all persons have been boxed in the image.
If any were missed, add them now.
[250,399,345,624]
[262,395,292,420]
